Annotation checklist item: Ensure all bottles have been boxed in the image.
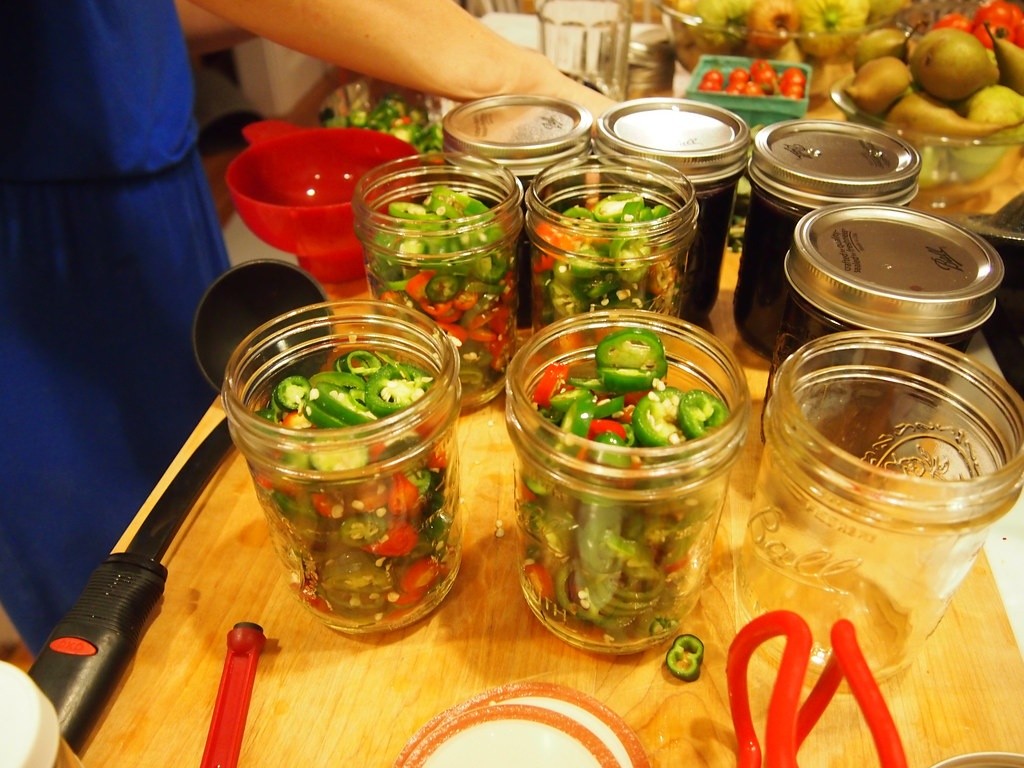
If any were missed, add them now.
[0,660,85,768]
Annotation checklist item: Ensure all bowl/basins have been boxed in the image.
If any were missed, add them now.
[653,0,916,97]
[226,120,420,283]
[831,73,1024,205]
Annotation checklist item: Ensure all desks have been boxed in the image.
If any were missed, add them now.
[30,246,1024,768]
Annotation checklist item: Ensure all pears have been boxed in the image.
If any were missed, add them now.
[844,23,1024,190]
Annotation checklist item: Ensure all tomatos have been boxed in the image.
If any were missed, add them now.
[925,0,1024,51]
[696,58,806,100]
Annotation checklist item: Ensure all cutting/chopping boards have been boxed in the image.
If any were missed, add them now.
[74,249,1024,768]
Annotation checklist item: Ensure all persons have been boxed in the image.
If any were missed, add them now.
[0,1,621,662]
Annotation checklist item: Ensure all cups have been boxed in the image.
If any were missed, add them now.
[354,152,524,411]
[733,120,922,360]
[443,94,593,328]
[506,309,751,656]
[536,0,632,103]
[594,97,749,329]
[221,299,463,634]
[761,204,1004,441]
[524,155,700,335]
[737,330,1024,683]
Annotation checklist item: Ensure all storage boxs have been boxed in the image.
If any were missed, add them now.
[688,53,813,123]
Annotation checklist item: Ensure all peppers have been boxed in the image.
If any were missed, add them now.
[516,324,729,637]
[666,634,705,682]
[368,188,682,404]
[324,97,447,163]
[244,346,455,623]
